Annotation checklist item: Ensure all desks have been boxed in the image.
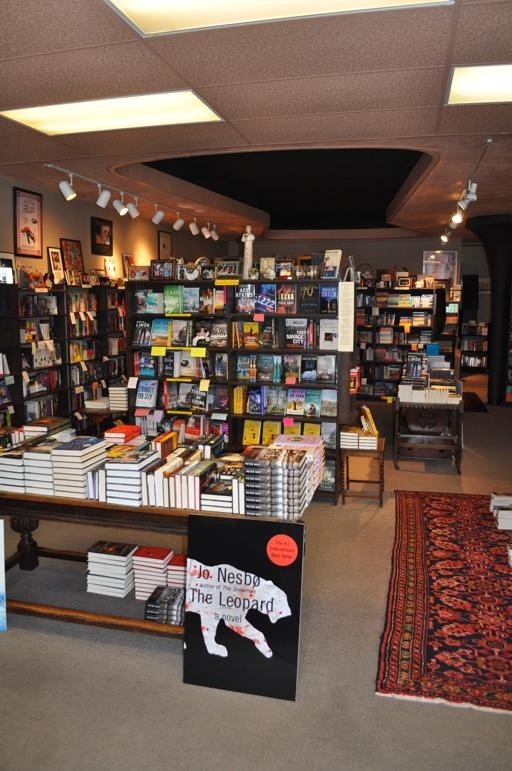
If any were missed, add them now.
[0,489,293,641]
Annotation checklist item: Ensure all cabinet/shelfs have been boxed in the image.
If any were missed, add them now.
[0,257,491,507]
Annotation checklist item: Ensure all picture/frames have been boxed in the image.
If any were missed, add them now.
[156,228,173,260]
[57,237,85,274]
[46,244,64,283]
[11,183,44,259]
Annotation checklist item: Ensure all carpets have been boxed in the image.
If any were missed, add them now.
[373,487,512,715]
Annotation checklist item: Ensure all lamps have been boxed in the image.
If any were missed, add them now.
[57,168,221,242]
[439,177,479,244]
